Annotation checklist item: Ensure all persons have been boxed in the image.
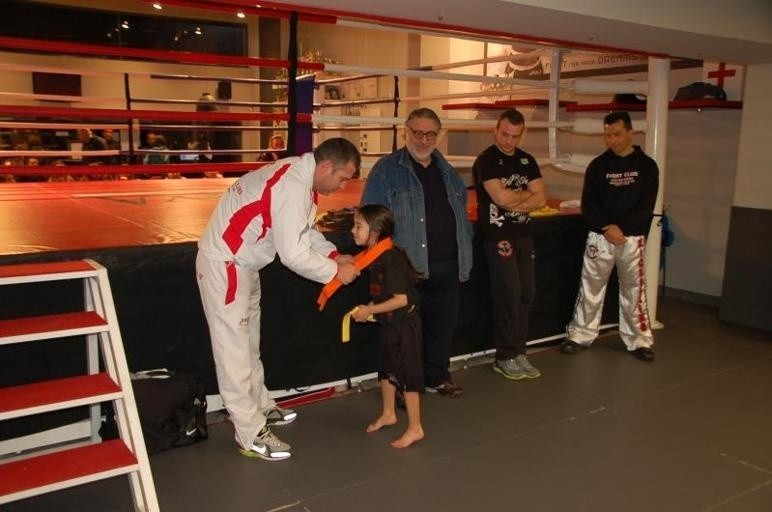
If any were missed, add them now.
[557,107,659,360]
[350,205,426,450]
[1,124,283,183]
[361,106,474,398]
[192,137,360,465]
[471,109,548,381]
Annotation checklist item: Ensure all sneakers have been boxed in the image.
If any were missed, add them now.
[630,348,654,361]
[263,406,297,425]
[494,354,541,380]
[561,339,585,354]
[423,378,464,397]
[236,424,294,461]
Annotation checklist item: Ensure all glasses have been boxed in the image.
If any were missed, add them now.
[408,124,437,140]
